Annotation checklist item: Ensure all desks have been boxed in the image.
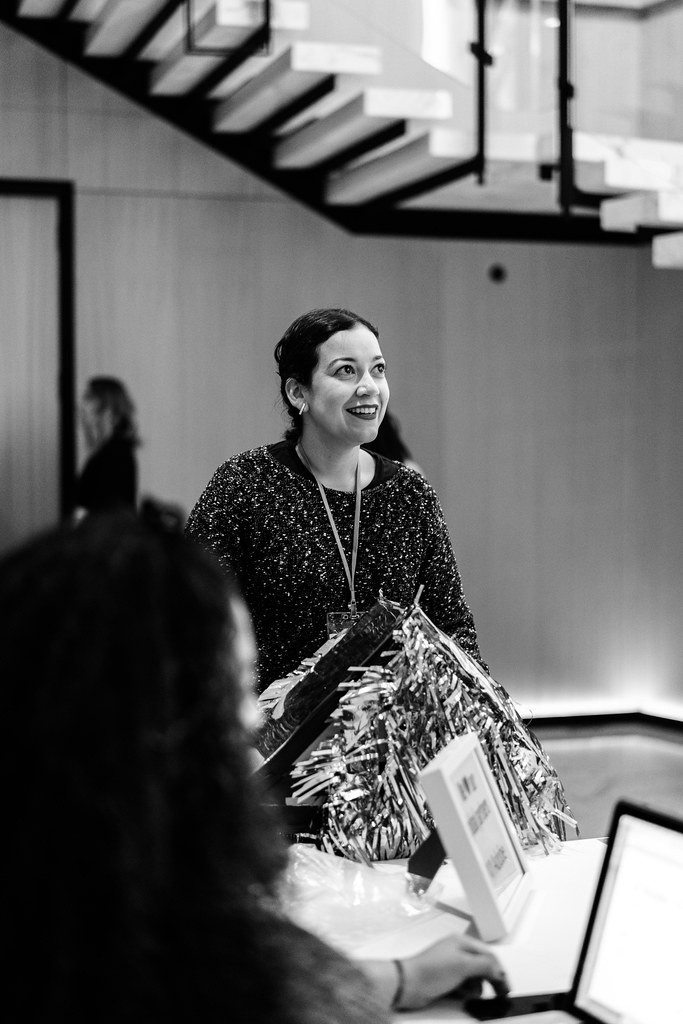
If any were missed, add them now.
[341,834,617,1024]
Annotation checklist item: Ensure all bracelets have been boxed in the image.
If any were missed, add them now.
[387,956,406,1012]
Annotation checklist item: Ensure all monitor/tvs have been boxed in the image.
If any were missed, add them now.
[563,801,682,1024]
[251,604,398,776]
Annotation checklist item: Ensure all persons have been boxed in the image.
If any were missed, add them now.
[0,505,512,1024]
[71,373,143,529]
[185,307,492,761]
